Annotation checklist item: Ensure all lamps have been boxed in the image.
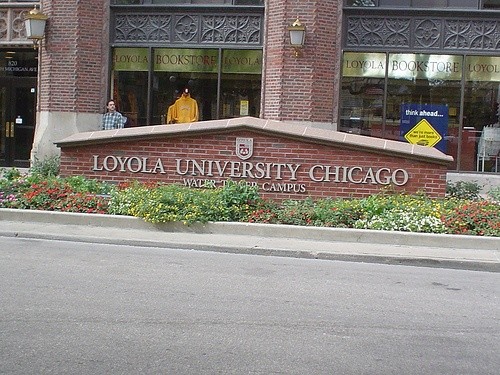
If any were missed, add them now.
[287,12,308,58]
[23,5,47,51]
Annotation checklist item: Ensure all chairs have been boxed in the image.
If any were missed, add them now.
[476,126,500,173]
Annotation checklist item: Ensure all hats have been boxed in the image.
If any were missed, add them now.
[183,86,190,94]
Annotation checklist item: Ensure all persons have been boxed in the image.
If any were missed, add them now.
[101,99,124,130]
